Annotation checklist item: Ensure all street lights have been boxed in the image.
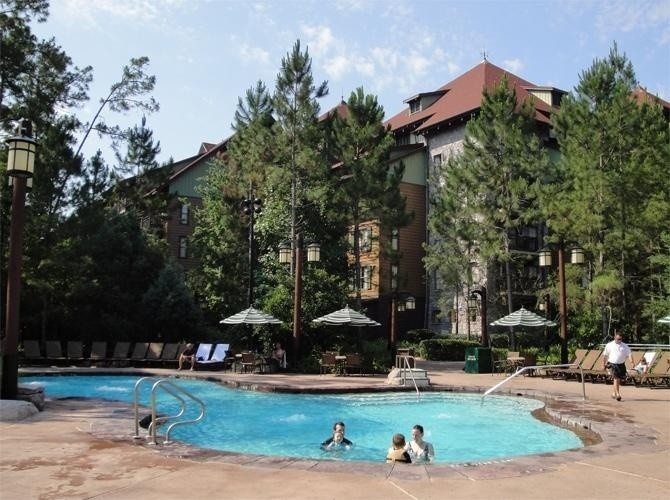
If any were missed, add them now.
[535,235,586,367]
[276,232,320,368]
[390,292,416,354]
[243,193,261,306]
[0,115,45,422]
[467,285,489,347]
[538,291,551,352]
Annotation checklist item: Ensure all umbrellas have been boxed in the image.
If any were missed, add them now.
[657,315,670,323]
[489,305,557,356]
[219,304,284,350]
[312,304,382,355]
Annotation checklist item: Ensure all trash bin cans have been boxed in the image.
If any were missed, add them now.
[465,347,491,374]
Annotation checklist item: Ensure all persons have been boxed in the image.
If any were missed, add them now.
[320,422,352,450]
[327,432,350,451]
[404,425,434,464]
[265,342,284,365]
[386,434,411,463]
[626,357,648,377]
[178,343,196,371]
[603,332,635,401]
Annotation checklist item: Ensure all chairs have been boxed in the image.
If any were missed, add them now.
[194,343,213,367]
[86,341,108,366]
[45,341,66,365]
[122,342,149,366]
[104,342,130,367]
[198,344,230,367]
[152,343,179,365]
[66,341,85,365]
[22,338,45,365]
[225,349,287,374]
[135,342,163,366]
[489,346,670,390]
[167,344,194,365]
[312,349,365,375]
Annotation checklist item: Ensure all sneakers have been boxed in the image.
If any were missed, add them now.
[611,391,616,399]
[616,394,622,401]
